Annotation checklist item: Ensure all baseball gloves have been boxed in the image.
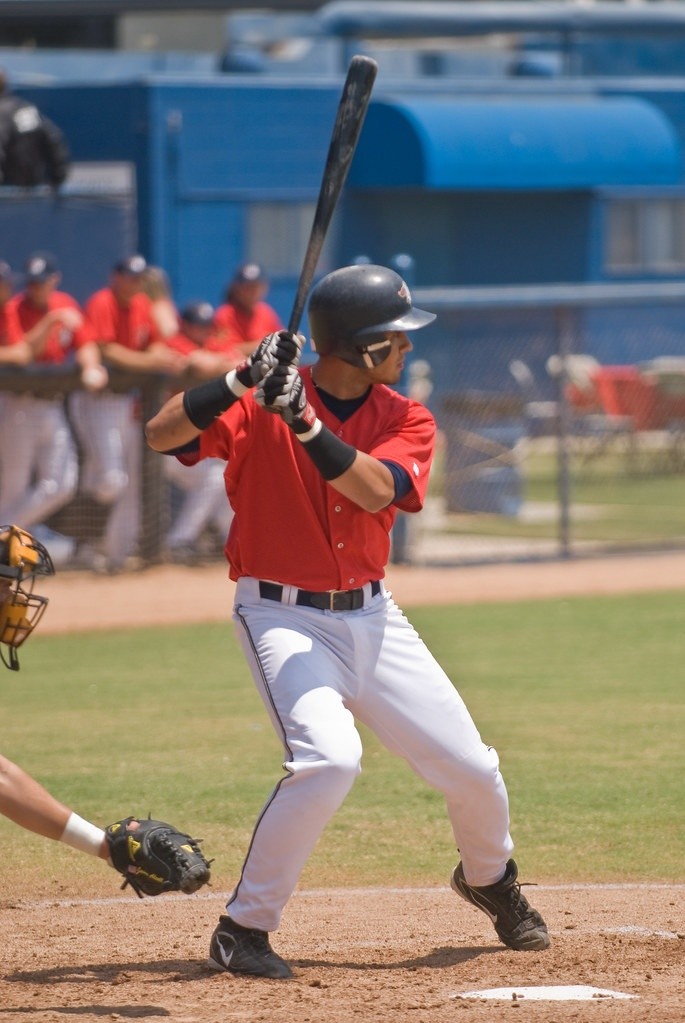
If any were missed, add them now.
[105,816,211,896]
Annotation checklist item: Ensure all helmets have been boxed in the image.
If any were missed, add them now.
[307,261,440,372]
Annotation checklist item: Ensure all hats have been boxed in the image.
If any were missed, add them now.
[27,249,59,284]
[115,250,150,277]
[238,262,267,284]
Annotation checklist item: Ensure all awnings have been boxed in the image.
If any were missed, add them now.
[348,95,684,192]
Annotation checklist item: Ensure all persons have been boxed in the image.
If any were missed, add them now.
[0,251,109,577]
[160,563,160,565]
[64,256,217,575]
[558,353,685,475]
[157,302,216,496]
[154,265,295,564]
[144,264,550,979]
[0,524,212,897]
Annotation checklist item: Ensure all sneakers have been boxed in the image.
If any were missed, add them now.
[450,858,552,953]
[207,914,291,982]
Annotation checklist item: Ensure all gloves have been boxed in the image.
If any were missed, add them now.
[235,326,308,390]
[254,365,316,434]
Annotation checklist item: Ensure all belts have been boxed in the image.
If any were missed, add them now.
[259,578,382,613]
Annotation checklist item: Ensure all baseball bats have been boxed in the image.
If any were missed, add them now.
[262,54,378,413]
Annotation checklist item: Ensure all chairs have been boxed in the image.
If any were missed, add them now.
[441,355,684,507]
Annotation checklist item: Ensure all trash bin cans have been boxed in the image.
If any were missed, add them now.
[444,393,527,514]
